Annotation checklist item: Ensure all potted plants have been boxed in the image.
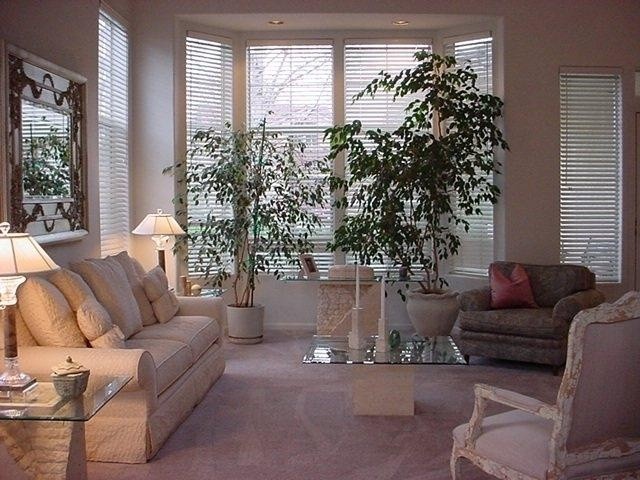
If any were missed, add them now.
[161,110,332,344]
[321,48,512,340]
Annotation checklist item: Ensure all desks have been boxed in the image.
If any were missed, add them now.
[278,275,428,339]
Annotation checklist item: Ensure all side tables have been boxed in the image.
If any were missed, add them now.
[0,372,135,479]
[167,286,230,299]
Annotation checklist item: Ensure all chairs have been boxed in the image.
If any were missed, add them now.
[457,261,605,376]
[450,291,640,480]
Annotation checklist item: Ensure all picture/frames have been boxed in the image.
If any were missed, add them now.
[297,254,321,279]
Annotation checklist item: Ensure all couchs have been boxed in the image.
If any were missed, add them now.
[1,257,229,464]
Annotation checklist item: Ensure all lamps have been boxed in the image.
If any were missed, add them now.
[131,207,186,274]
[0,222,61,396]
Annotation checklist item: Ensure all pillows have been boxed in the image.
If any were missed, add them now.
[17,250,181,349]
[489,262,542,311]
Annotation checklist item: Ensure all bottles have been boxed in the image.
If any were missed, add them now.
[389,328,401,348]
[185,282,191,297]
[179,276,186,295]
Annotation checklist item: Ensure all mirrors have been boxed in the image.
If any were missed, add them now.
[0,39,90,245]
